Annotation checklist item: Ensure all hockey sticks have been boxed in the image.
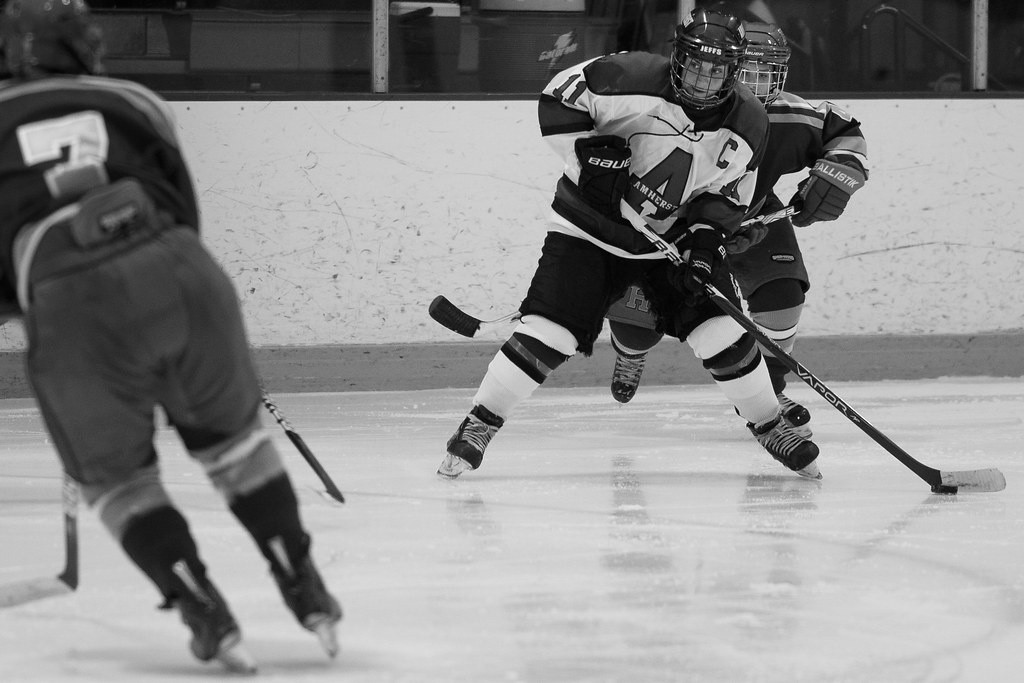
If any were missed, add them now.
[0,472,80,607]
[620,198,1006,494]
[428,201,802,342]
[258,390,350,509]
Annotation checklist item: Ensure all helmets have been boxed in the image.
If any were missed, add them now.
[0,0,103,77]
[741,24,793,109]
[669,6,747,114]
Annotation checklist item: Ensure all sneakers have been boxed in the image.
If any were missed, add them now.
[776,392,813,442]
[285,583,343,655]
[611,352,647,406]
[746,404,824,482]
[181,580,254,674]
[436,405,504,482]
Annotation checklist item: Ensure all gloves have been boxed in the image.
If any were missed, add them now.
[667,249,720,311]
[785,174,848,227]
[578,144,632,221]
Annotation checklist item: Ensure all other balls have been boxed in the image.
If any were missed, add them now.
[930,483,959,495]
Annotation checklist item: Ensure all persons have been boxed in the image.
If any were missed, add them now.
[0,0,343,669]
[435,4,823,485]
[609,19,869,438]
